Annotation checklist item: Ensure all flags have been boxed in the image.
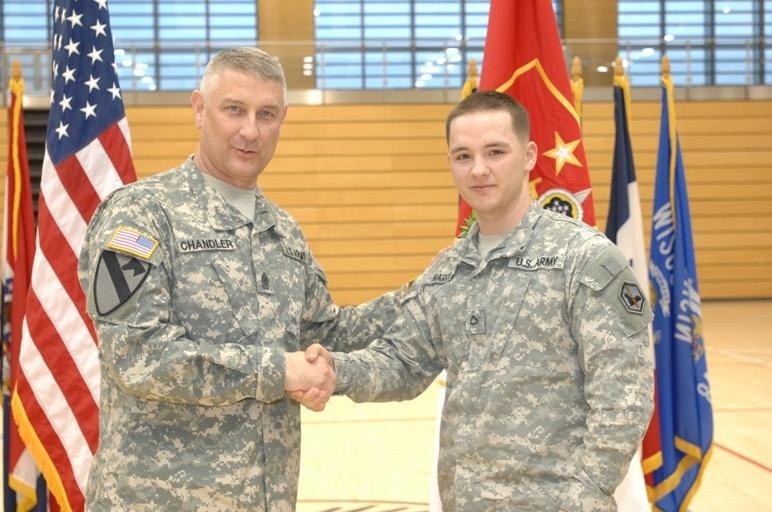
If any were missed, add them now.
[457,0,596,236]
[7,0,138,512]
[635,53,714,512]
[453,1,595,236]
[1,59,35,404]
[605,57,650,310]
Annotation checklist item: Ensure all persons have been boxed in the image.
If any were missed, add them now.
[77,47,452,512]
[288,90,655,512]
